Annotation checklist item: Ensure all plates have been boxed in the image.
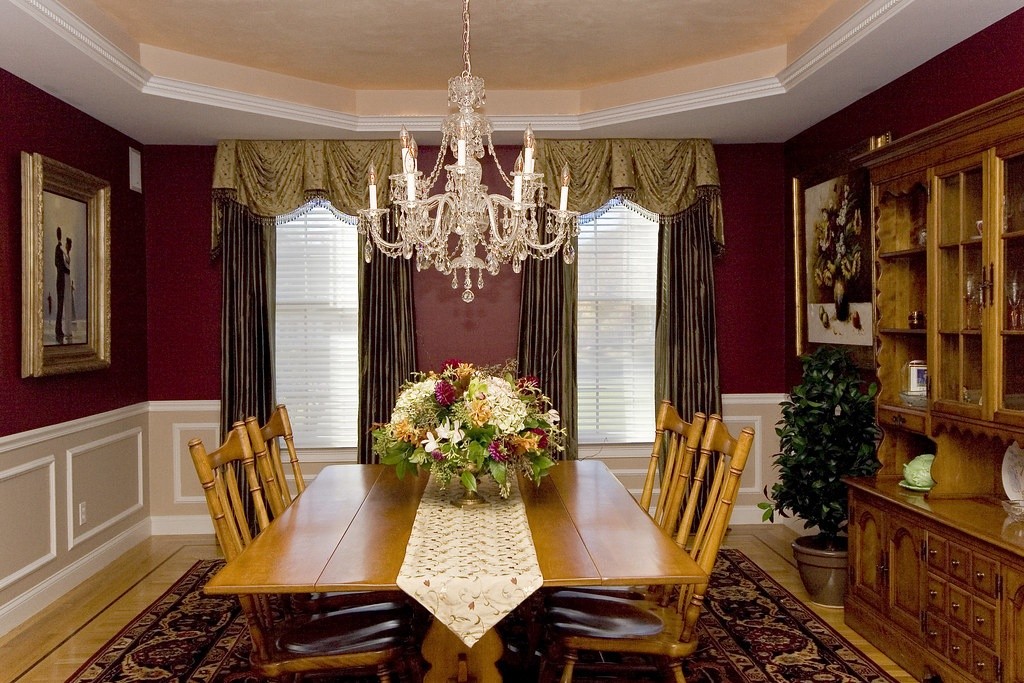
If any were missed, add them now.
[971,236,982,239]
[1002,441,1024,501]
[899,480,932,491]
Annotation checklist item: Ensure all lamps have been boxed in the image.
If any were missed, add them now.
[358,0,581,304]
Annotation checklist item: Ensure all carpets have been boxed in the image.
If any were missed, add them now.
[61,560,899,682]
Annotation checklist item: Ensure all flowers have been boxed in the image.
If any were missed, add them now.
[365,357,569,499]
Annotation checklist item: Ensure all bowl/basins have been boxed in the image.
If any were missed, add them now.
[1002,501,1024,525]
[899,391,928,407]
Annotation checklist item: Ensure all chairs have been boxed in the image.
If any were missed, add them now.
[540,400,705,598]
[188,419,406,682]
[245,404,407,612]
[536,414,755,682]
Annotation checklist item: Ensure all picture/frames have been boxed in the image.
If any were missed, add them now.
[908,362,927,393]
[791,131,892,363]
[20,152,112,378]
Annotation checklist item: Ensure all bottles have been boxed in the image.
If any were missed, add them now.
[1008,282,1024,330]
[908,361,928,391]
[967,273,983,329]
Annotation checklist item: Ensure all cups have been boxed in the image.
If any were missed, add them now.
[977,221,983,235]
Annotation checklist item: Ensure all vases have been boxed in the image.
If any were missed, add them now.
[451,464,492,511]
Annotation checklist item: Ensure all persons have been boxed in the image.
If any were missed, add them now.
[55,227,72,344]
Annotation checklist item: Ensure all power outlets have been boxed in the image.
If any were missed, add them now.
[79,501,87,526]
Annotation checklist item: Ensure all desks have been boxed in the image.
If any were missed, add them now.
[202,458,709,682]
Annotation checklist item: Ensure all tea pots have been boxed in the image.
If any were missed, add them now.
[904,454,935,486]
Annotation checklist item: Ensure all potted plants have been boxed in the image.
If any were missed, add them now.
[757,347,885,608]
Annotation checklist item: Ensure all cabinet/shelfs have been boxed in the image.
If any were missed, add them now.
[841,82,1024,683]
